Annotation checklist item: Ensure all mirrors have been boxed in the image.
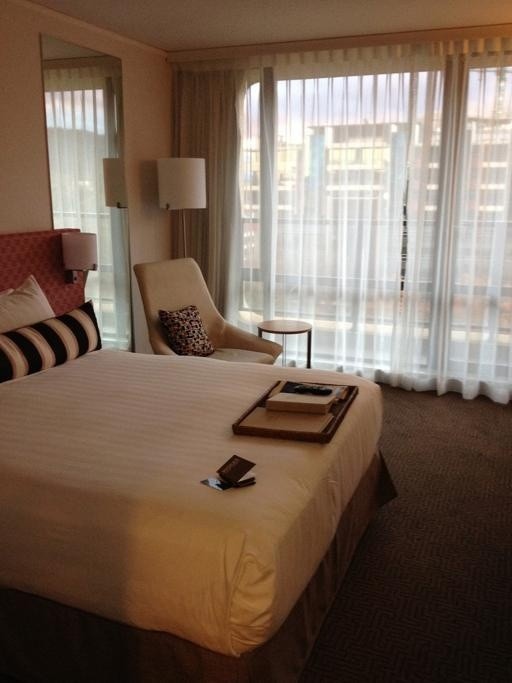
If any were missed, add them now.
[40,33,135,351]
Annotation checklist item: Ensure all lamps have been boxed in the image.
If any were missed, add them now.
[157,155,207,257]
[102,155,128,208]
[61,230,99,287]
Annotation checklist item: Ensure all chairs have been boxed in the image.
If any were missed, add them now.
[133,259,284,365]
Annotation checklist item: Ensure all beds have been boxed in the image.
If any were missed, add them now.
[1,227,383,682]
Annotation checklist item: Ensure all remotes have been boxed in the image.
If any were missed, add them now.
[293,384,333,396]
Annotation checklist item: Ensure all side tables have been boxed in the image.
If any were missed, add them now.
[257,319,314,369]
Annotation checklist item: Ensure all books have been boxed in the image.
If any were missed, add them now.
[263,380,341,415]
[216,453,257,488]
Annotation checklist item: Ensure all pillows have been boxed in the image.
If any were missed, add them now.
[157,303,214,357]
[1,274,103,385]
